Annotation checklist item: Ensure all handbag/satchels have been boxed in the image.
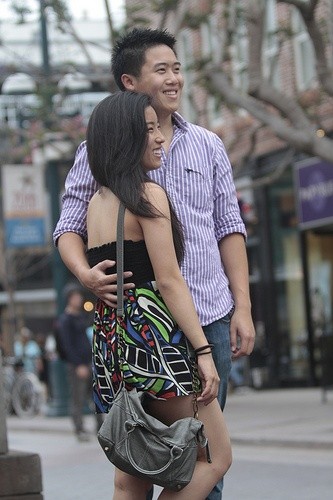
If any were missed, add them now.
[97,381,213,492]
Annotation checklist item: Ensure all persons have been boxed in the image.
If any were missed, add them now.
[13,326,100,420]
[53,28,257,499]
[233,316,333,396]
[54,282,108,439]
[85,92,233,500]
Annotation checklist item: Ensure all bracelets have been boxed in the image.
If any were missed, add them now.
[195,344,216,353]
[196,350,213,358]
